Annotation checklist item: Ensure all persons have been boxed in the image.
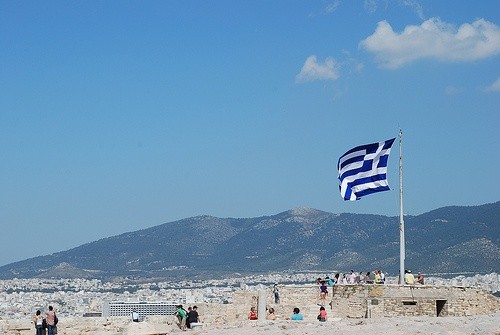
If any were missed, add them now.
[292,308,303,320]
[248,307,256,320]
[320,282,327,306]
[174,305,198,331]
[35,310,43,335]
[318,268,424,285]
[267,308,275,320]
[274,282,279,303]
[319,307,327,321]
[44,306,55,335]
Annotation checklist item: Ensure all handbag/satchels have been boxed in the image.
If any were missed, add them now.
[54,312,58,325]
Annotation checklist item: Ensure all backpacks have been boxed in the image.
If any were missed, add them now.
[42,319,47,328]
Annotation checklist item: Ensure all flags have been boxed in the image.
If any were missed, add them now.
[337,137,402,201]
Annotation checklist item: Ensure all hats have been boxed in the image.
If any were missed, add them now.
[193,306,198,309]
